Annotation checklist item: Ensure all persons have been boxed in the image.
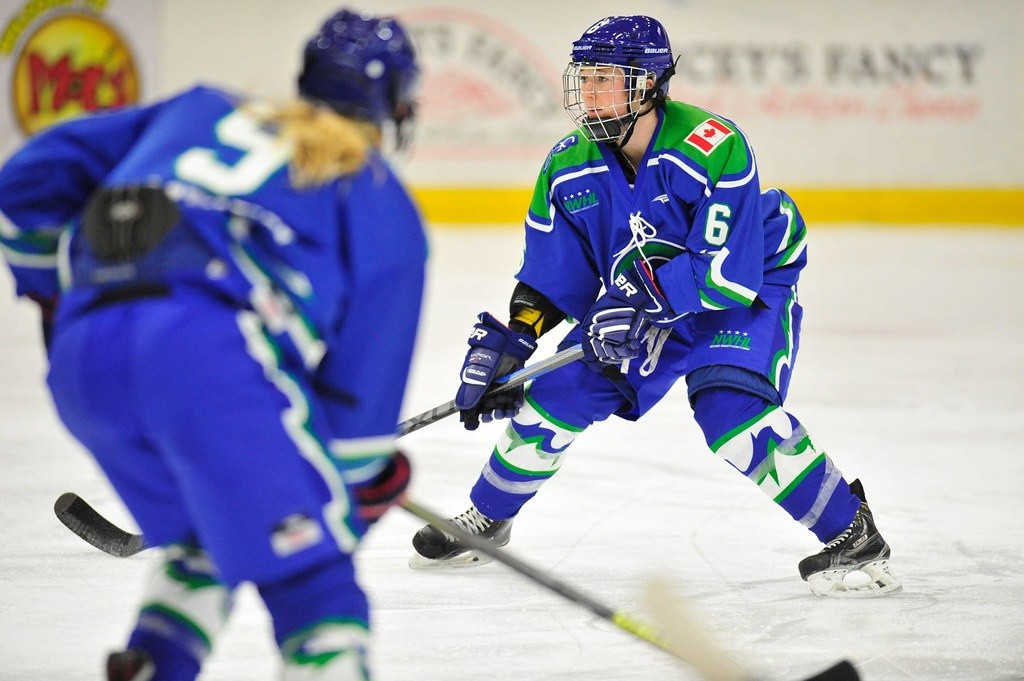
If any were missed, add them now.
[411,13,904,599]
[0,5,431,680]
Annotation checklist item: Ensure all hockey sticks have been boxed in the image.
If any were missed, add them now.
[397,500,860,681]
[54,344,584,558]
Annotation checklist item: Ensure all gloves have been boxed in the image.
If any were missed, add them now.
[455,311,537,429]
[582,259,678,371]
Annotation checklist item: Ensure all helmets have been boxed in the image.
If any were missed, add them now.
[298,7,420,150]
[561,14,681,141]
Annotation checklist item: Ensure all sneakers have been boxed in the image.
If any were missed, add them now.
[407,506,512,569]
[798,477,902,598]
